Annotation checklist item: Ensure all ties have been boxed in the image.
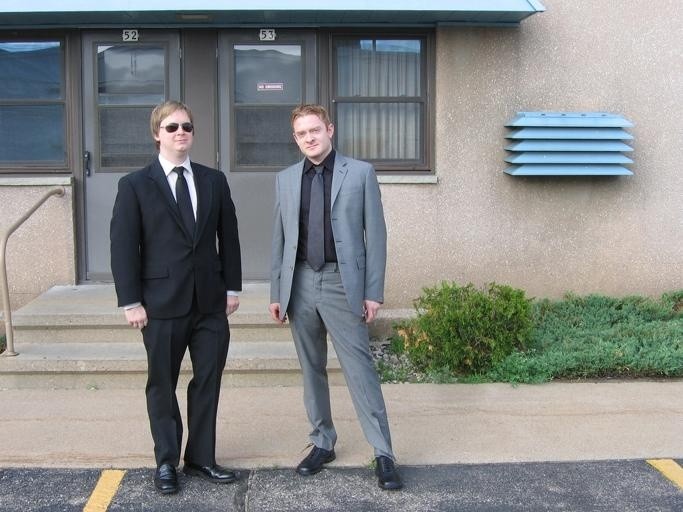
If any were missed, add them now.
[173,167,195,240]
[307,165,326,273]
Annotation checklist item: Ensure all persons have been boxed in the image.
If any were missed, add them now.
[110,99,242,496]
[264,104,407,493]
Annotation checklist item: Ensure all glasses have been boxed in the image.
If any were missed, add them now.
[161,122,193,133]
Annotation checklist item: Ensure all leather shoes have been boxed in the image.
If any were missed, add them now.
[296,445,336,475]
[154,463,177,494]
[375,456,402,489]
[183,462,236,483]
